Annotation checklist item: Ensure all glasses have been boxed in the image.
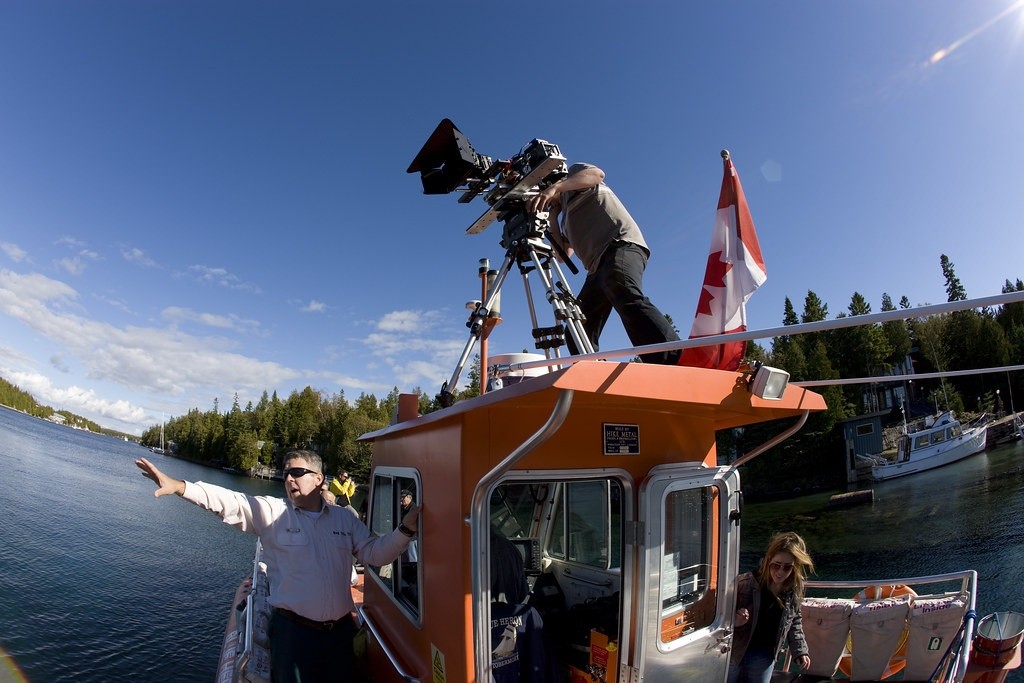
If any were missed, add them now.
[320,489,324,491]
[282,467,318,481]
[342,474,348,479]
[768,562,795,573]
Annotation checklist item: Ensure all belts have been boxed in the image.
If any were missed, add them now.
[278,608,351,630]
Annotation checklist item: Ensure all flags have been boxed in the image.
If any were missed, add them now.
[676,158,768,372]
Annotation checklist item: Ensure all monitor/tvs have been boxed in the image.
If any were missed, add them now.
[506,537,542,573]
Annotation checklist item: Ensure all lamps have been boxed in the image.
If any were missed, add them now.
[740,358,789,401]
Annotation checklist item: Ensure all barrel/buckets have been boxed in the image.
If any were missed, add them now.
[971,612,1024,668]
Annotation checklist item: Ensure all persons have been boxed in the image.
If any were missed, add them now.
[135,450,422,683]
[728,531,814,683]
[320,481,336,505]
[400,489,416,515]
[490,522,529,605]
[330,470,356,501]
[526,162,684,366]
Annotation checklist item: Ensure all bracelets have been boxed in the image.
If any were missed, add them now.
[398,523,415,536]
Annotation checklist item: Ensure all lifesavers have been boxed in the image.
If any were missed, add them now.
[838,585,920,680]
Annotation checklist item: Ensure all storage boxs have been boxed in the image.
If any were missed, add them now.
[566,627,617,683]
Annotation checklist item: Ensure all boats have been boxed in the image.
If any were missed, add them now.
[855,409,989,483]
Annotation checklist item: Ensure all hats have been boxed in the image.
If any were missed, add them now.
[401,489,413,496]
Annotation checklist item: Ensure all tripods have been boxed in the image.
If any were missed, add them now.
[434,202,598,408]
